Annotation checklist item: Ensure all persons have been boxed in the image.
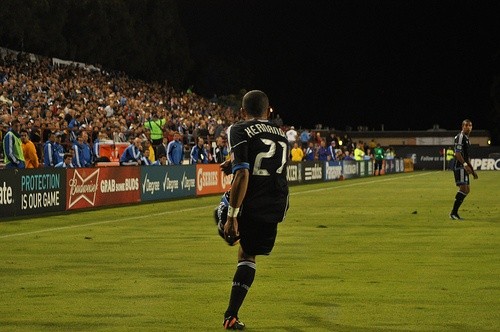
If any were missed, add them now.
[273,114,397,161]
[1,47,251,171]
[215,90,290,329]
[446,145,455,171]
[373,143,385,176]
[449,119,479,220]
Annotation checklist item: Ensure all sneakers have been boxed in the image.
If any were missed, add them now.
[223,316,246,330]
[450,213,462,220]
[215,194,228,237]
